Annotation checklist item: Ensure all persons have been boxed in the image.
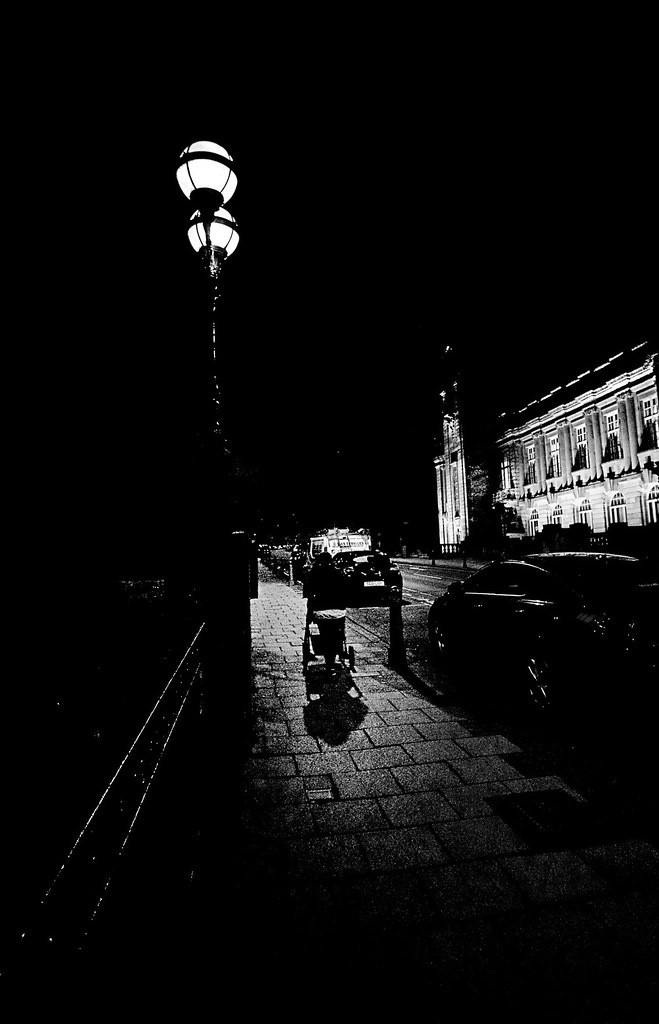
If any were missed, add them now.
[303,547,347,639]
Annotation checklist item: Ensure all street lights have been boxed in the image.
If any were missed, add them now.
[177,137,248,502]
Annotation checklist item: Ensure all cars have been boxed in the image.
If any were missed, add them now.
[256,535,405,606]
[427,551,659,682]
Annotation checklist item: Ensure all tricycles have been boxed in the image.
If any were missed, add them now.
[300,564,355,678]
